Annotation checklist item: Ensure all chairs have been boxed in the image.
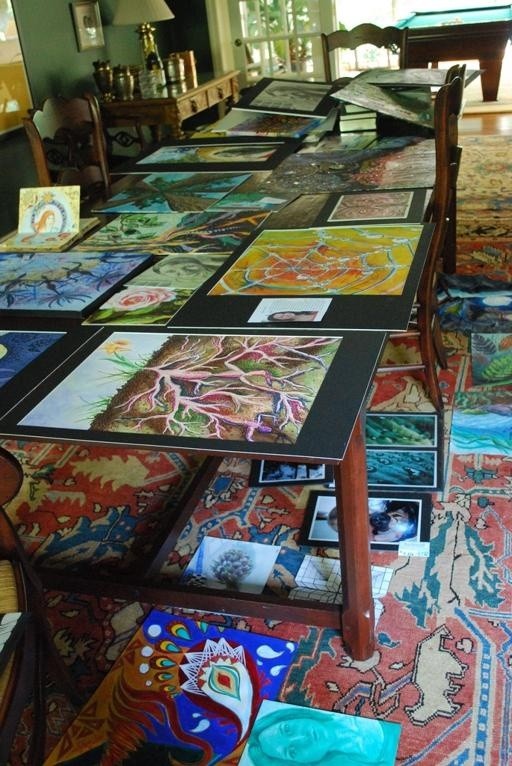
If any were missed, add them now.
[374,55,466,412]
[320,22,408,85]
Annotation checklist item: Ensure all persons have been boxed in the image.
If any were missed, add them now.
[152,255,222,288]
[370,506,412,544]
[267,310,318,321]
[83,15,92,38]
[87,17,95,37]
[244,707,400,766]
[263,83,327,112]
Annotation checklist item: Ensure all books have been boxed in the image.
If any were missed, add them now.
[1,185,101,253]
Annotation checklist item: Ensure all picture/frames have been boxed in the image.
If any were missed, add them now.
[0,0,36,141]
[71,0,105,53]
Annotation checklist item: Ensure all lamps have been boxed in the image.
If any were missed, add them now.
[111,0,176,87]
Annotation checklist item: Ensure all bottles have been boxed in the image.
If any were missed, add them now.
[91,60,134,101]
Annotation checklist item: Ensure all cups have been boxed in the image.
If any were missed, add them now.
[138,73,159,97]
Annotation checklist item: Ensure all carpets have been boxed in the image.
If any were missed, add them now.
[460,85,512,114]
[0,128,511,766]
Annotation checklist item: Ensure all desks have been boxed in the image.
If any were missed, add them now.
[391,4,512,101]
[0,69,429,660]
